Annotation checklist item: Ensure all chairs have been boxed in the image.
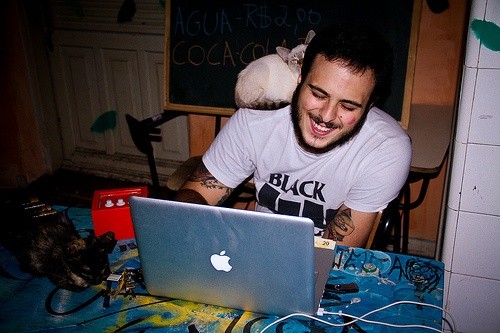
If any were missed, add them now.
[169,147,410,253]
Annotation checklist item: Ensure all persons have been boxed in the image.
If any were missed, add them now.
[177,20,412,249]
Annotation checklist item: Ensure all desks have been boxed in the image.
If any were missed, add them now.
[0,206,445,333]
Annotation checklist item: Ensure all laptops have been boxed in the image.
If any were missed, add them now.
[128,194,336,320]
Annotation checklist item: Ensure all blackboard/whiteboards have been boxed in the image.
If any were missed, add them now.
[162,0,421,130]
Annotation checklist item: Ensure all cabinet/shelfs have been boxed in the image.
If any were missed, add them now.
[45,0,192,189]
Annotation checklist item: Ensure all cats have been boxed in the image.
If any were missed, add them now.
[234,29,316,111]
